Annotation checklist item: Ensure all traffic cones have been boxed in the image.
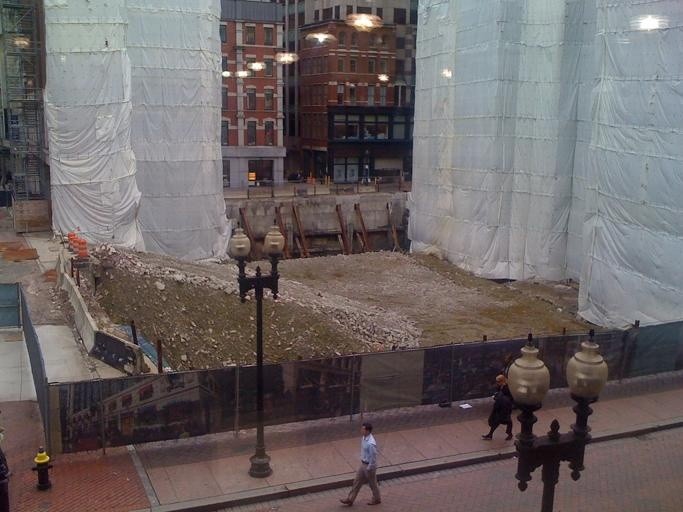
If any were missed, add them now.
[65,231,93,262]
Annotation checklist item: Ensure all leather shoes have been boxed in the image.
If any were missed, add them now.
[506,435,513,440]
[340,499,353,505]
[367,499,381,505]
[482,434,492,438]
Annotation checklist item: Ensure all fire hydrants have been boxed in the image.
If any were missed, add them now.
[31,444,55,492]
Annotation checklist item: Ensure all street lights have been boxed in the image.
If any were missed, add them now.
[506,325,610,512]
[226,214,288,480]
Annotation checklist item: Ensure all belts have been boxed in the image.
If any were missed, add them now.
[361,459,369,464]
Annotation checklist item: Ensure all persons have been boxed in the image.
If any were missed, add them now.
[481,374,520,441]
[340,423,381,506]
[1,430,13,512]
